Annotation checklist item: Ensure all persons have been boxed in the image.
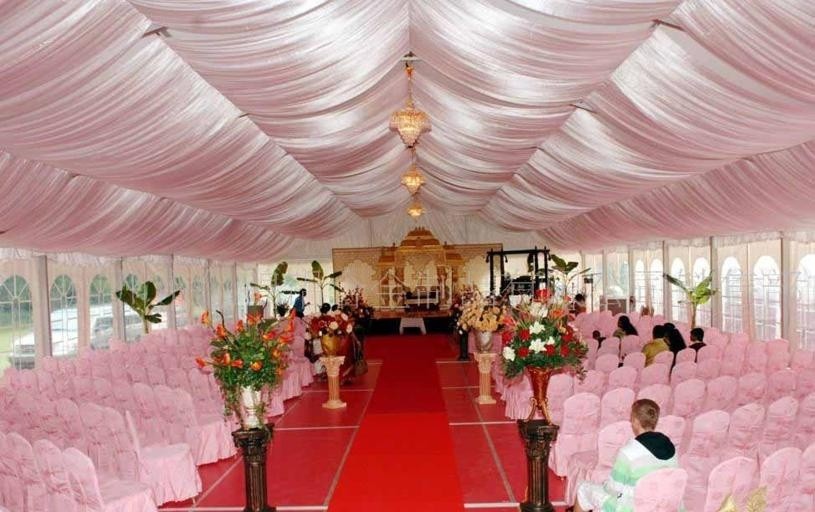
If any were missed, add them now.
[564,398,679,512]
[303,301,364,364]
[291,288,311,319]
[574,293,583,316]
[579,293,586,315]
[592,315,708,376]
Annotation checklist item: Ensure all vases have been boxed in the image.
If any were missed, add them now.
[320,333,339,357]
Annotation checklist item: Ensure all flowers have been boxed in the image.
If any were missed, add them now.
[310,311,354,337]
[456,301,501,332]
[196,291,297,429]
[500,290,589,386]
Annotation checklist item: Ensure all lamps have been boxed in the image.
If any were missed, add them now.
[389,57,431,221]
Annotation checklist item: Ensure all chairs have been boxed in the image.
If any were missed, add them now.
[467,310,815,512]
[0,313,321,511]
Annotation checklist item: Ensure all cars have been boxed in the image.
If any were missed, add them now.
[8,303,144,368]
